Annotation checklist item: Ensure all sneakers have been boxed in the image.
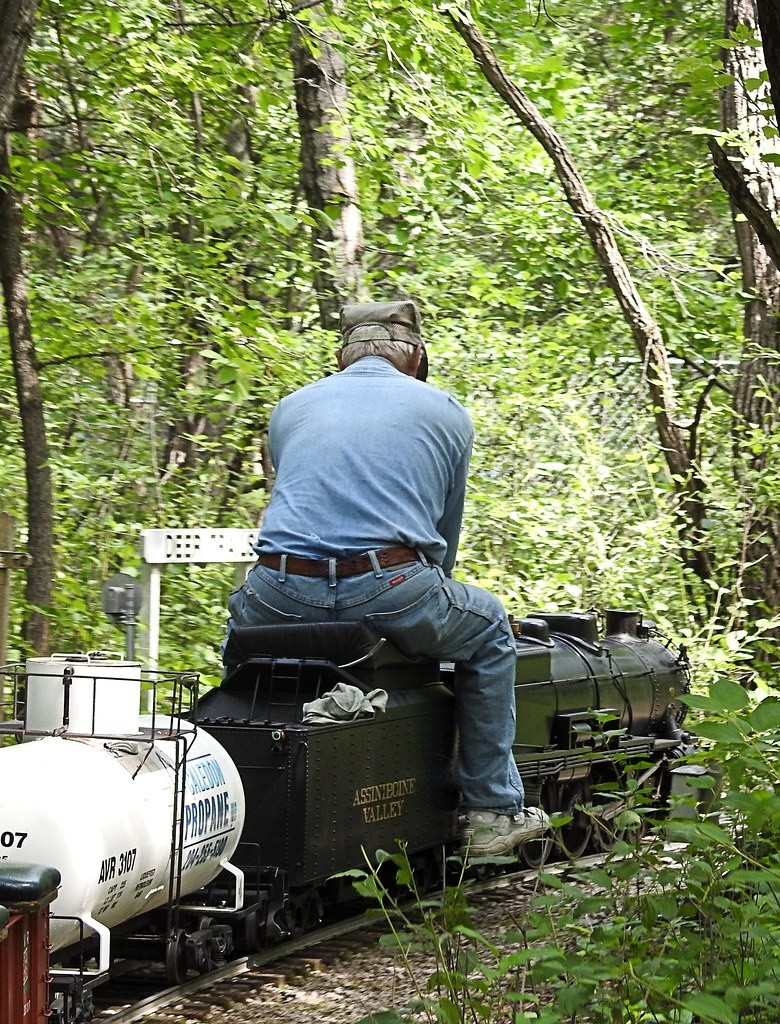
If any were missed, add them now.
[457,806,550,858]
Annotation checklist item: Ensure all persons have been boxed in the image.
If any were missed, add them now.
[223,300,550,860]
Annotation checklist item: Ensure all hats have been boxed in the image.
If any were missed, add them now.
[338,300,428,382]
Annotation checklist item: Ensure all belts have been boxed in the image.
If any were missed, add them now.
[259,545,429,578]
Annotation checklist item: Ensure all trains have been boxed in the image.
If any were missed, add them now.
[0,565,704,1023]
